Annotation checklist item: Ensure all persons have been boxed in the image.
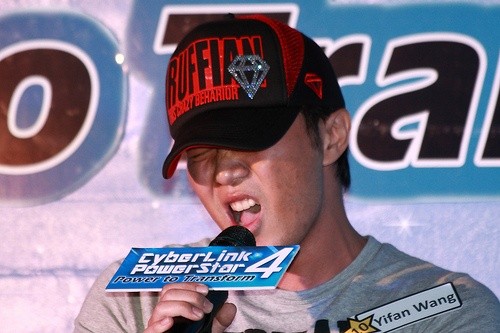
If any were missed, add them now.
[72,14,500,333]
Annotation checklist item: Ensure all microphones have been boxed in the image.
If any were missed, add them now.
[106,224,301,333]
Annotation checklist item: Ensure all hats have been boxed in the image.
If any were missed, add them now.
[162,12,343,180]
